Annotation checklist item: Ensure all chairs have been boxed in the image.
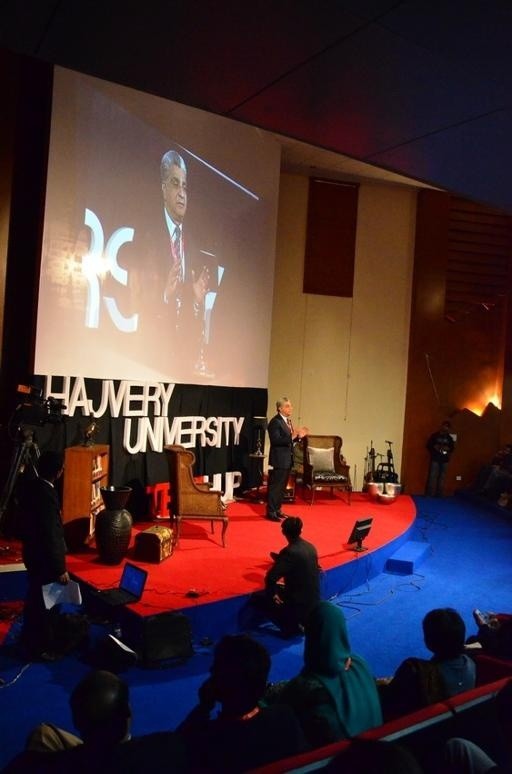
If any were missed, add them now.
[243,653,512,774]
[163,444,229,548]
[301,435,354,507]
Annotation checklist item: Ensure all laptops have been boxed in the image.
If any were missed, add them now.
[91,563,147,607]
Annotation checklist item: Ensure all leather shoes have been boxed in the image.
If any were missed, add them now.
[277,510,290,518]
[267,511,282,521]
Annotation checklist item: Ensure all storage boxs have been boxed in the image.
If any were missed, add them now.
[136,525,176,565]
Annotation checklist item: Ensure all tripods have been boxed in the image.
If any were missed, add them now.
[0,424,40,520]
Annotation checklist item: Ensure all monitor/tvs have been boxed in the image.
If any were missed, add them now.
[347,518,373,552]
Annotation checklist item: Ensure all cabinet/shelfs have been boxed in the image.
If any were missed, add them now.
[64,444,111,543]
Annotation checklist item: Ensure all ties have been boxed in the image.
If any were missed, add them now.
[287,418,293,434]
[174,225,184,325]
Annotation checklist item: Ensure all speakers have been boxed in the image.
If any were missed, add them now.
[303,177,360,297]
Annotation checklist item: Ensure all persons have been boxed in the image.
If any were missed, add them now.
[142,150,211,364]
[23,448,70,660]
[267,397,295,521]
[425,419,512,510]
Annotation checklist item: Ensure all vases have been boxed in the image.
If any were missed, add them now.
[95,484,133,565]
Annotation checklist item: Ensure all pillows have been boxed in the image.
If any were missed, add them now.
[306,446,336,473]
